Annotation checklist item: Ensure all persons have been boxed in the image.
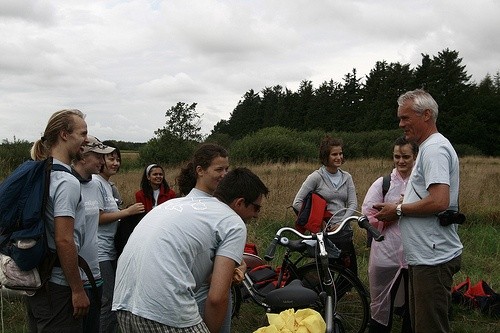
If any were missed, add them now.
[136,163,178,213]
[176,143,247,333]
[25,108,91,333]
[71,135,116,333]
[292,138,358,302]
[373,89,463,333]
[112,168,269,333]
[91,140,145,333]
[362,135,420,333]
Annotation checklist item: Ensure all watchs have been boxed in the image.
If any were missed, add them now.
[396,204,402,215]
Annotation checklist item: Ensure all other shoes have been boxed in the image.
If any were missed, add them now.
[0,253,42,297]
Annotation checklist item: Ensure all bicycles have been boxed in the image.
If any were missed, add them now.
[232,208,385,333]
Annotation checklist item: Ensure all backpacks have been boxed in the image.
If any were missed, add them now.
[113,204,147,259]
[0,156,54,271]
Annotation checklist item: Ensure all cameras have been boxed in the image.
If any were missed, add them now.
[439,210,465,226]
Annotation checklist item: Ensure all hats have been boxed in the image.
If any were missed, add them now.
[78,134,116,155]
